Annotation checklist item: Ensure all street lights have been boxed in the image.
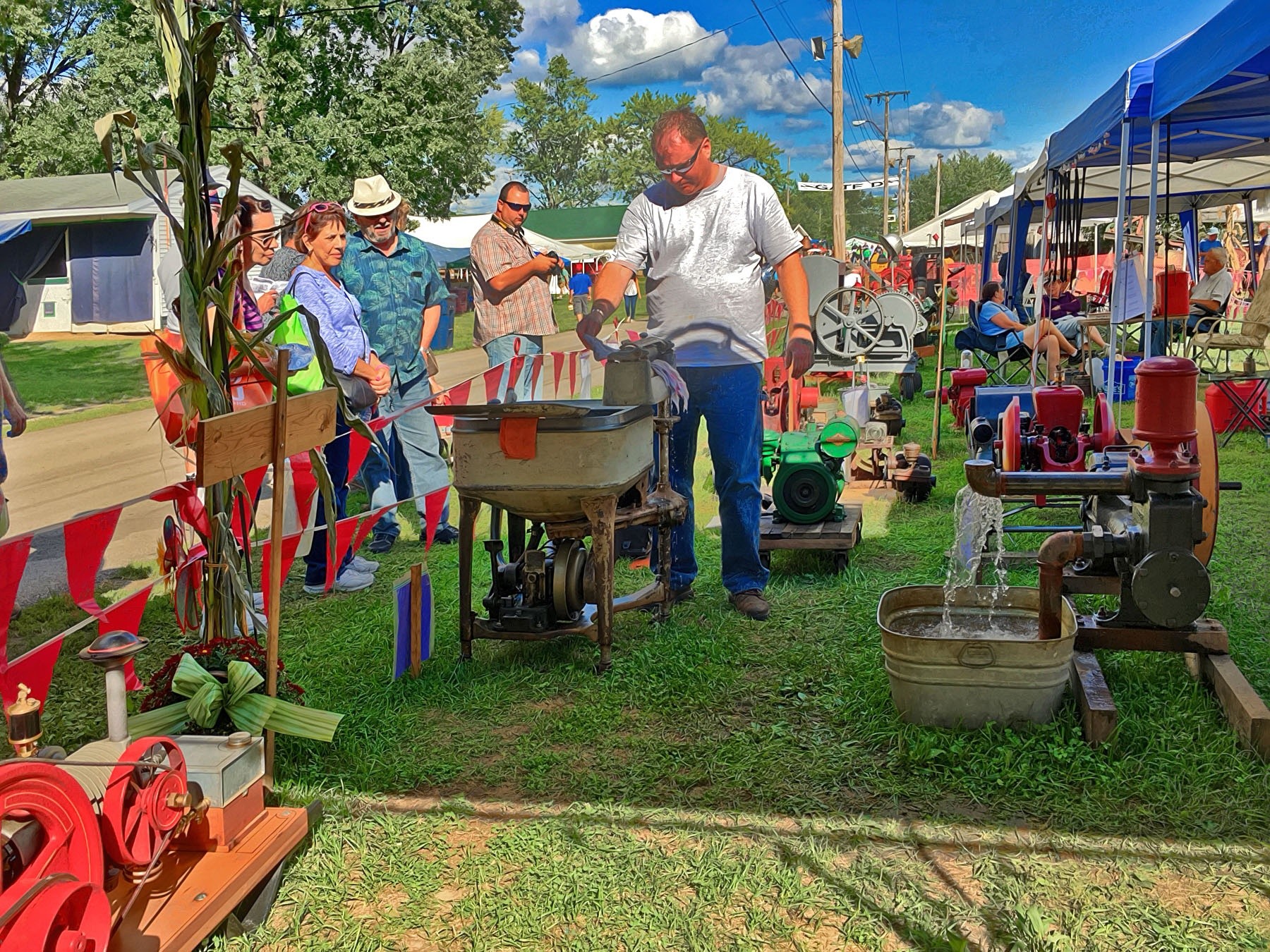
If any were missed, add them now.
[934,153,944,218]
[852,120,890,236]
[904,154,915,234]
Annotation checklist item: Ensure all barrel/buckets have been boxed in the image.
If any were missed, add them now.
[1102,356,1142,401]
[1156,264,1191,317]
[877,585,1079,729]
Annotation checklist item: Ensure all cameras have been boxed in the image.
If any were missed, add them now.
[546,251,562,277]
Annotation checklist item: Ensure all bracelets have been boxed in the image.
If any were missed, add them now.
[374,364,388,370]
[592,308,605,322]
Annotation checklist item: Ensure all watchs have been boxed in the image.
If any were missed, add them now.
[420,346,429,356]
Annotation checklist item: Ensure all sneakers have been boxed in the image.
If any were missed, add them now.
[1068,349,1097,365]
[302,569,374,594]
[1047,379,1057,386]
[1102,343,1119,355]
[345,556,379,573]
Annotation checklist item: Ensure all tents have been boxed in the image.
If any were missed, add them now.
[391,0,1270,460]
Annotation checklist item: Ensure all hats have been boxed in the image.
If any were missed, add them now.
[575,263,582,270]
[1043,275,1060,284]
[348,174,402,216]
[1207,227,1219,235]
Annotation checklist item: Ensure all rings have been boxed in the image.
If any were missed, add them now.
[386,387,390,393]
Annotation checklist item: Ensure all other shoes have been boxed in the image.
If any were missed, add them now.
[637,586,694,612]
[11,602,22,620]
[243,590,264,610]
[367,534,396,554]
[419,522,459,544]
[732,589,769,620]
[200,604,267,639]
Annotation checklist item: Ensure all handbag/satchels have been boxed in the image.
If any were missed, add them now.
[323,369,378,412]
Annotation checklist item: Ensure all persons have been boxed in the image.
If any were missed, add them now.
[1,133,1270,611]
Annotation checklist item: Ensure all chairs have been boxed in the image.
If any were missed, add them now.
[955,300,1051,385]
[1190,291,1230,377]
[1075,270,1113,313]
[1187,270,1270,386]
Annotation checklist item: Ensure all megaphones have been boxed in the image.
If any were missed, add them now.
[888,158,896,168]
[810,36,827,61]
[843,35,863,59]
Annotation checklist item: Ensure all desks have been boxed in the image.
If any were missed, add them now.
[1077,315,1190,399]
[1209,370,1270,448]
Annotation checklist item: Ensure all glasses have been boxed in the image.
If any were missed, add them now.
[656,137,704,175]
[249,230,284,245]
[305,202,343,233]
[500,198,531,211]
